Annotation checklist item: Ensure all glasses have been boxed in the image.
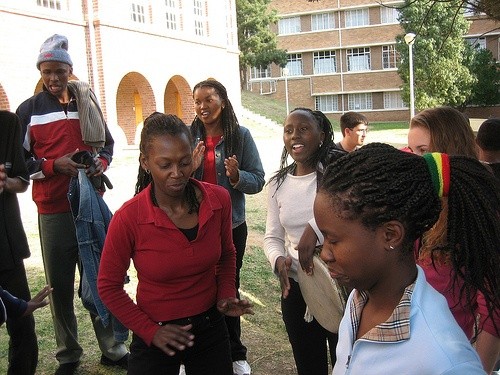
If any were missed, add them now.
[350,128,368,136]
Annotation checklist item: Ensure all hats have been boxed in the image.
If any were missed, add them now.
[35,33,74,70]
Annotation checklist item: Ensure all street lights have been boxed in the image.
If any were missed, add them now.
[403,32,416,119]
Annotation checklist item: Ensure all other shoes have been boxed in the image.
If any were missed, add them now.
[54,360,81,375]
[100,351,131,370]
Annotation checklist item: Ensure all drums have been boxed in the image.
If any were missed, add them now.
[296,244,354,333]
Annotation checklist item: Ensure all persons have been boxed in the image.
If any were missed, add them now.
[400,107,500,375]
[263,108,339,375]
[314,143,500,375]
[189,80,265,375]
[16,34,130,375]
[0,111,54,375]
[97,112,256,375]
[323,112,369,168]
